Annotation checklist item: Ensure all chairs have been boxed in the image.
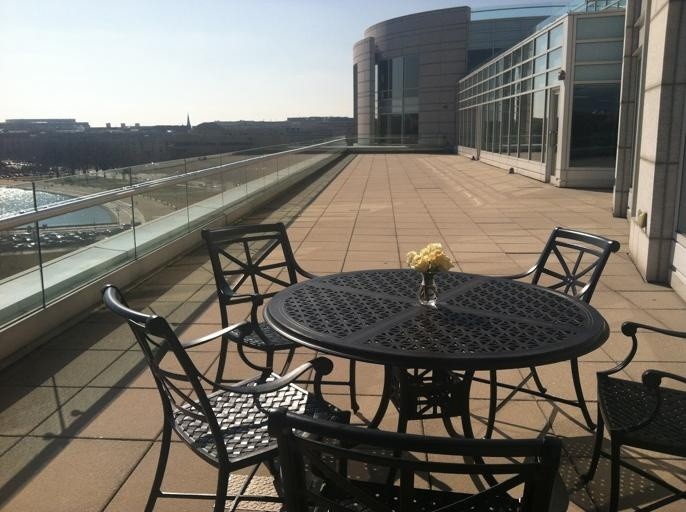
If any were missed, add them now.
[258,404,561,511]
[452,224,622,439]
[198,219,363,422]
[574,316,686,511]
[100,284,353,510]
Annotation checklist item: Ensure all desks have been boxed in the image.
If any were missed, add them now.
[259,268,612,510]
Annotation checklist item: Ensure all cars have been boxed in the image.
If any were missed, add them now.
[0,219,140,252]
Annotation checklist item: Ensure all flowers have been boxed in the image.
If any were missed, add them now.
[404,242,456,275]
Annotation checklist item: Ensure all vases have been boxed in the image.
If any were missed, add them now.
[418,271,439,307]
